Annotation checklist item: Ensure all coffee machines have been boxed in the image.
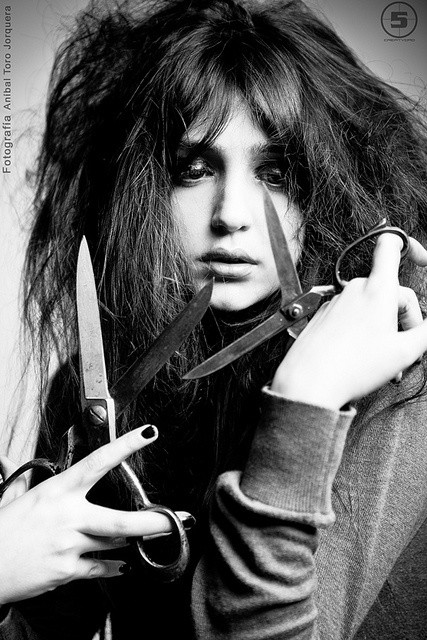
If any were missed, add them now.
[1,233,215,587]
[182,181,412,381]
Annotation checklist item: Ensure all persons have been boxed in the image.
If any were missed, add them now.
[1,0,427,640]
